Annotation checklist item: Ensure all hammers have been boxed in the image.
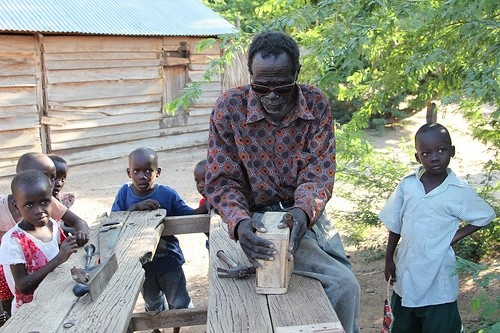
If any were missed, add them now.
[82,244,100,271]
[216,250,256,279]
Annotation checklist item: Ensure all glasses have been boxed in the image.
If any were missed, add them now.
[249,70,297,96]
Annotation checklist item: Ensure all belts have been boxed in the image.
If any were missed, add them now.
[254,200,296,211]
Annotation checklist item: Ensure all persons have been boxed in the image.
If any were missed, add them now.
[49,156,67,202]
[111,147,213,333]
[0,152,90,321]
[194,159,207,206]
[0,169,78,316]
[378,122,496,333]
[205,32,361,333]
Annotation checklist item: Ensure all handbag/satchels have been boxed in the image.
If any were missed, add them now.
[381,276,394,333]
